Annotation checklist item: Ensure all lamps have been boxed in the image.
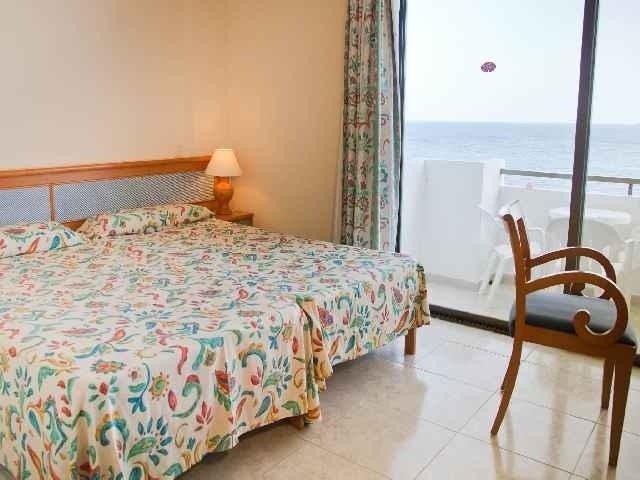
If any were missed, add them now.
[204,147,242,216]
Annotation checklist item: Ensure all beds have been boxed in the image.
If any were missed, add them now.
[0,155,428,478]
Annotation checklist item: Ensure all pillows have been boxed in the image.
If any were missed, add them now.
[1,204,217,259]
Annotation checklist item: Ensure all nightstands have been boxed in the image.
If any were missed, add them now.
[216,209,254,226]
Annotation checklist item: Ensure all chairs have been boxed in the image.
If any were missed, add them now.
[492,216,638,467]
[477,199,638,312]
